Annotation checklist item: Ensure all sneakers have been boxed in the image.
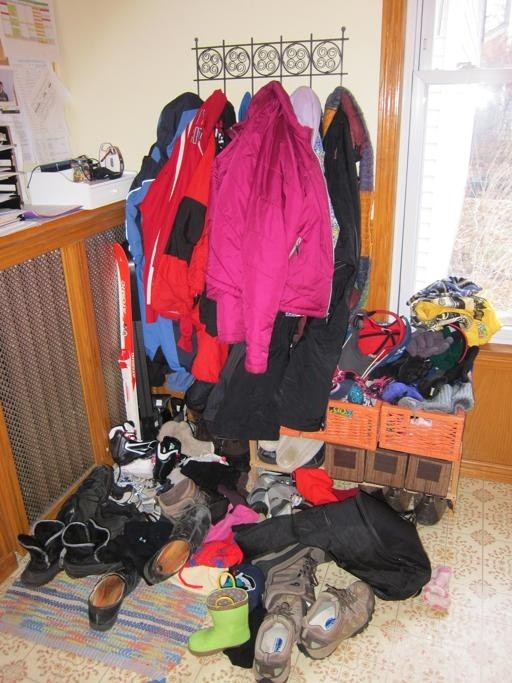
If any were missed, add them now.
[56,464,114,525]
[296,582,375,660]
[105,420,156,466]
[398,487,426,512]
[144,504,211,585]
[250,542,333,611]
[100,483,144,513]
[158,397,202,438]
[18,520,68,586]
[138,478,229,539]
[62,519,124,577]
[91,503,144,541]
[416,494,446,525]
[153,436,188,481]
[253,594,308,683]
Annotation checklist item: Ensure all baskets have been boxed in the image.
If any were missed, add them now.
[379,402,465,461]
[303,401,384,453]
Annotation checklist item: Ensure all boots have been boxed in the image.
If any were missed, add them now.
[188,572,250,656]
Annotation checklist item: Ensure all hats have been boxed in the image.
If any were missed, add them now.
[336,310,406,379]
[362,316,411,380]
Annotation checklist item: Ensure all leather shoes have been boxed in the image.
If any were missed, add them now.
[88,544,144,631]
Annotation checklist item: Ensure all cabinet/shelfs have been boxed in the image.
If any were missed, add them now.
[0,194,128,587]
